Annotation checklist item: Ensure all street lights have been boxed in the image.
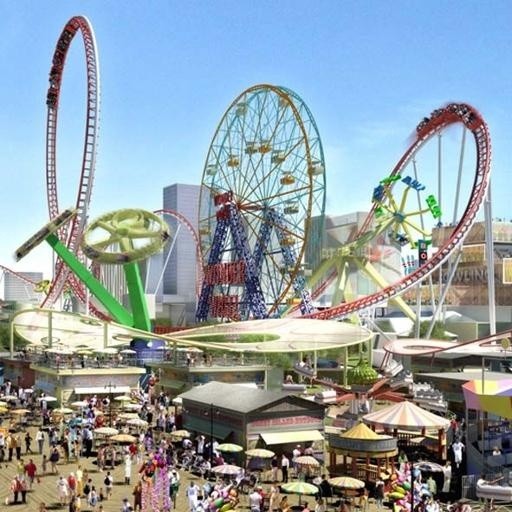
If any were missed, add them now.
[204,403,220,467]
[104,381,116,427]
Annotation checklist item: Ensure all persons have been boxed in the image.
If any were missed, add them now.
[424,104,473,124]
[1,349,501,512]
[47,31,71,106]
